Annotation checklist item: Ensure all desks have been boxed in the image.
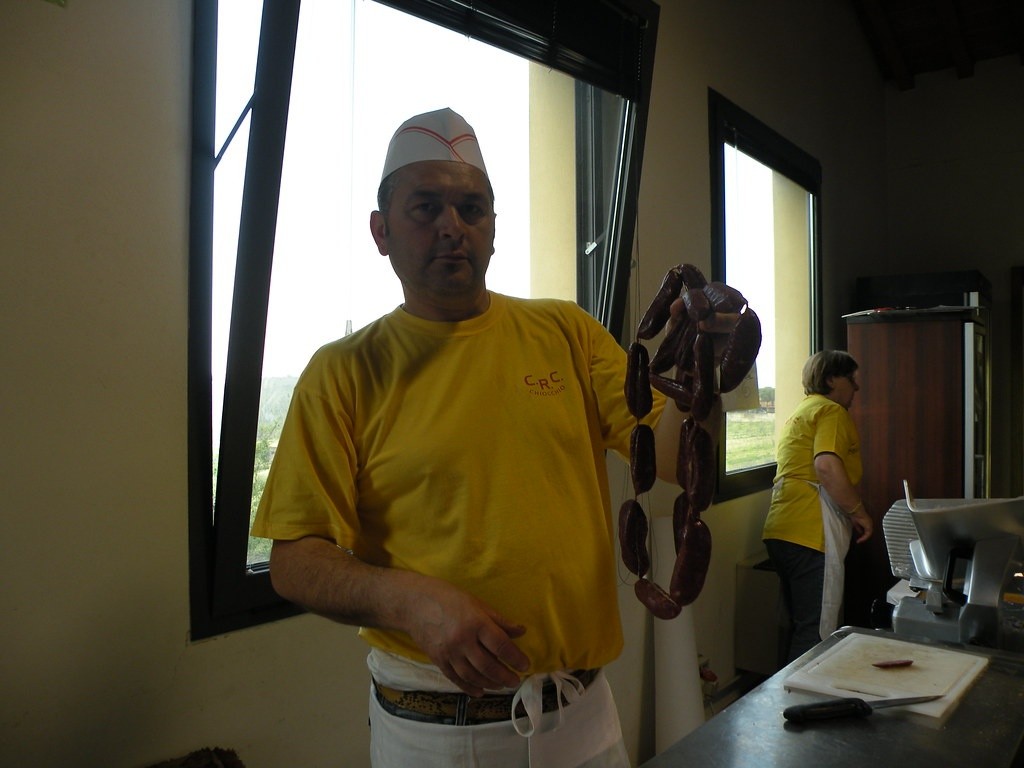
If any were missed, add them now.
[639,626,1024,768]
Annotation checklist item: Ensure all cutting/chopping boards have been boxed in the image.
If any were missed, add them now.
[785,632,989,730]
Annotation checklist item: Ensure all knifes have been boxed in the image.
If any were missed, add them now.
[783,695,945,721]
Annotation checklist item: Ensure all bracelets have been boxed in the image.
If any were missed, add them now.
[846,496,863,515]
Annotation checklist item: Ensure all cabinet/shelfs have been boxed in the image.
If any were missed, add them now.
[846,314,965,625]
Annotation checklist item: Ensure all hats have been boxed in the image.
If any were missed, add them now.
[379,107,489,182]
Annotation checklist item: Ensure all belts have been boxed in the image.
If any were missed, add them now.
[371,668,599,726]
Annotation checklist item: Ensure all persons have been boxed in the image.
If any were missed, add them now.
[760,348,874,669]
[247,106,741,768]
